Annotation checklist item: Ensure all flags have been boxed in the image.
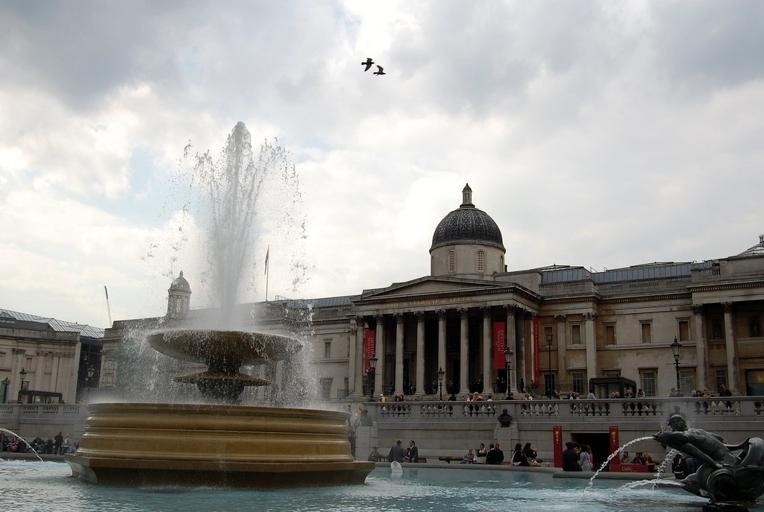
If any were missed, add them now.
[263,248,269,276]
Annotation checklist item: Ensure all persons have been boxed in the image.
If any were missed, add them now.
[375,393,387,415]
[368,446,380,462]
[445,383,743,420]
[653,414,743,469]
[348,425,357,460]
[394,391,404,416]
[561,440,594,472]
[406,439,418,463]
[0,431,78,456]
[357,404,370,426]
[388,440,407,462]
[620,449,652,465]
[346,404,352,428]
[383,376,538,394]
[459,441,543,468]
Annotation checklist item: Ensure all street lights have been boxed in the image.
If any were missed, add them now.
[669,336,683,396]
[368,353,378,402]
[87,364,95,393]
[545,334,554,400]
[17,368,28,403]
[437,367,446,409]
[504,346,514,400]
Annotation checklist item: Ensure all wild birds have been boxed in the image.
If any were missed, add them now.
[361,57,375,72]
[373,64,386,76]
